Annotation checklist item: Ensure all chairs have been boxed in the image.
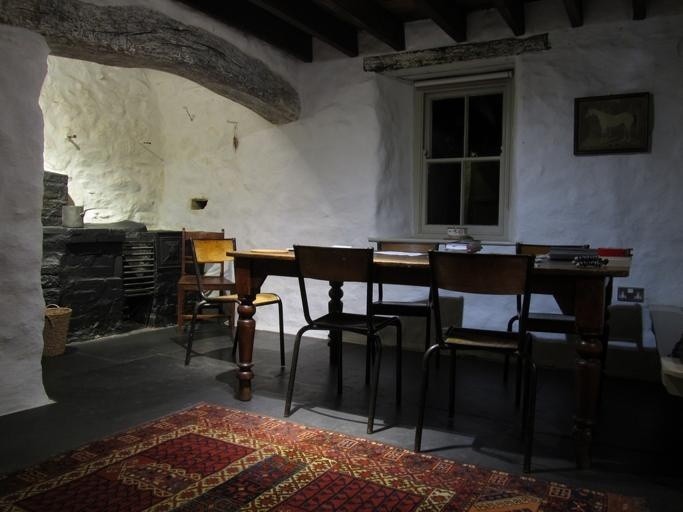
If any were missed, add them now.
[185,236,285,365]
[284,245,402,433]
[415,250,537,473]
[177,227,235,334]
[504,242,590,406]
[366,241,440,389]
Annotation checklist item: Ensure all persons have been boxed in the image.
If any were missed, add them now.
[582,98,634,151]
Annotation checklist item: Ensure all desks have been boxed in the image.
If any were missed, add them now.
[227,248,631,499]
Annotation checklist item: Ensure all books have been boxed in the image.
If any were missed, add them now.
[533,247,634,270]
[249,246,294,255]
[445,237,483,254]
[373,250,428,260]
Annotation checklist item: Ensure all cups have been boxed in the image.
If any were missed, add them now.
[60,205,84,228]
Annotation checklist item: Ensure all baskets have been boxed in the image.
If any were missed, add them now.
[44,304,72,357]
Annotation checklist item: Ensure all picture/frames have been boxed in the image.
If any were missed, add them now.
[574,92,650,155]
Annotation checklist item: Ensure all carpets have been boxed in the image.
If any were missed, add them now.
[0,400,651,512]
